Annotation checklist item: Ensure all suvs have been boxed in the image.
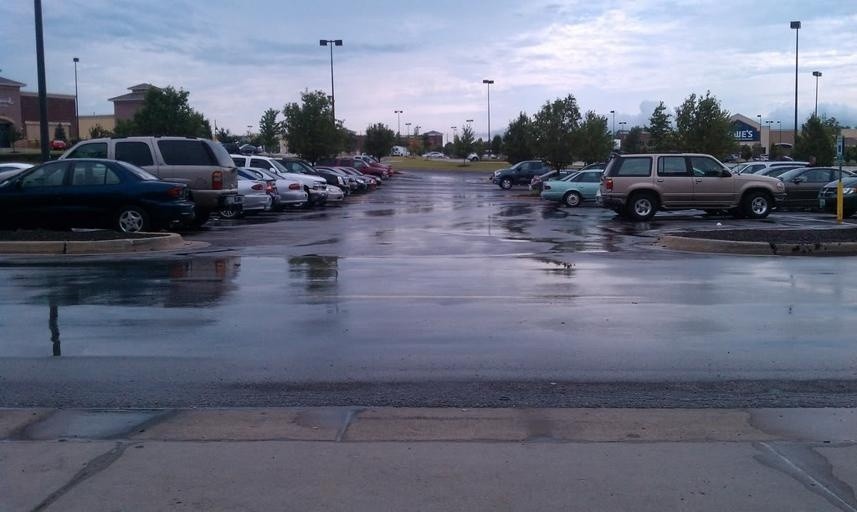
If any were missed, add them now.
[490,160,553,191]
[596,152,788,219]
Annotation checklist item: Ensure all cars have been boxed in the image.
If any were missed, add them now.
[49,140,66,149]
[423,151,446,159]
[816,174,857,217]
[530,160,608,207]
[468,150,499,162]
[0,158,196,233]
[771,167,857,211]
[219,140,396,219]
[0,162,43,186]
[724,161,810,179]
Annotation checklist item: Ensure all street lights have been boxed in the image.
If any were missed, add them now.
[405,122,422,137]
[610,111,627,138]
[73,57,80,141]
[483,79,495,157]
[757,112,782,153]
[813,70,822,118]
[790,21,801,144]
[319,39,343,127]
[451,119,474,136]
[394,110,403,131]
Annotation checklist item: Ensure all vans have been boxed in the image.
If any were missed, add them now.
[47,135,239,225]
[391,145,410,157]
